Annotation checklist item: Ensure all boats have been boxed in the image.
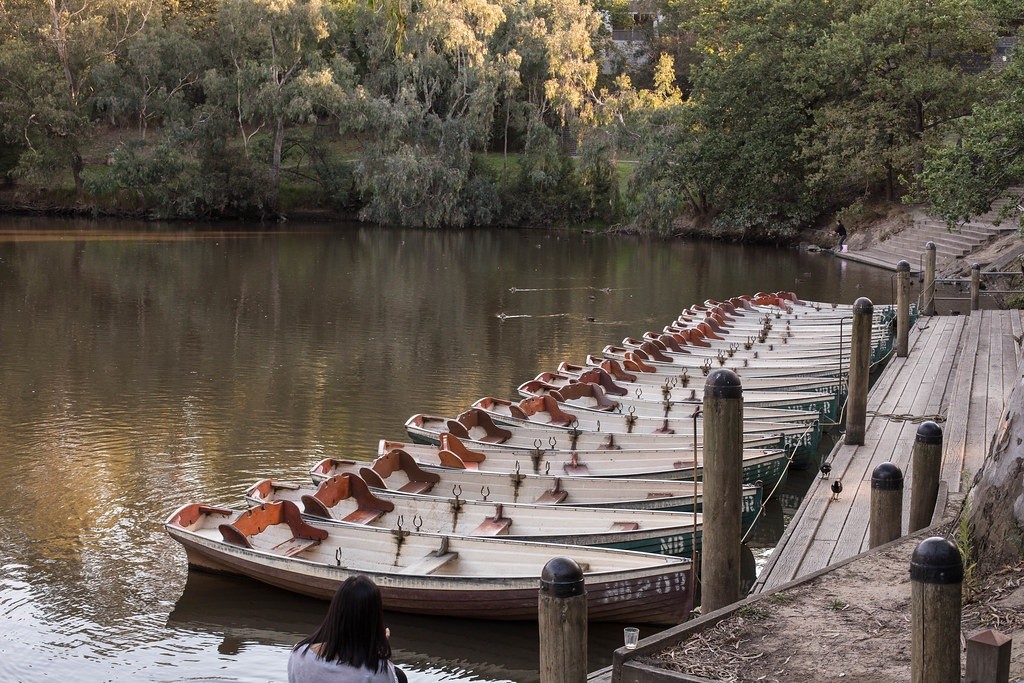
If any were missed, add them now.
[244,471,702,570]
[163,498,699,627]
[310,290,918,545]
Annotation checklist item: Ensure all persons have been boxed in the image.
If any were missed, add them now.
[833,220,847,252]
[287,576,408,683]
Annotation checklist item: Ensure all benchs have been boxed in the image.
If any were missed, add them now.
[578,562,589,573]
[509,395,577,427]
[447,408,511,445]
[361,448,441,494]
[634,342,673,362]
[569,367,628,397]
[623,352,656,373]
[607,523,638,530]
[218,499,328,558]
[301,472,394,526]
[535,490,569,504]
[438,432,487,470]
[468,518,512,535]
[652,334,691,353]
[549,382,619,412]
[672,291,806,347]
[597,398,702,500]
[600,359,637,383]
[399,551,458,574]
[564,463,593,477]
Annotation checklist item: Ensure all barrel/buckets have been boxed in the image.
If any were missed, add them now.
[842,245,848,253]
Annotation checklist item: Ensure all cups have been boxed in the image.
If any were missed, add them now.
[624,627,639,649]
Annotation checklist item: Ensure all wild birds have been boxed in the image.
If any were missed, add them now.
[831,481,843,501]
[818,462,832,480]
